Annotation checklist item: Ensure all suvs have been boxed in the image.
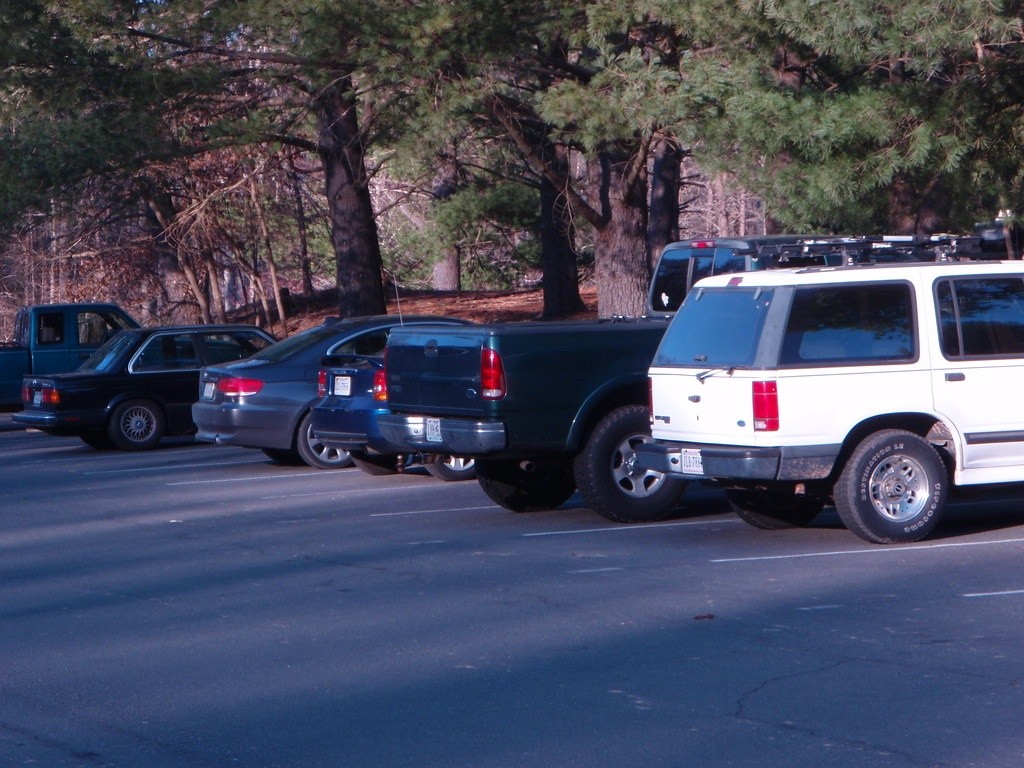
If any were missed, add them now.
[633,237,1024,546]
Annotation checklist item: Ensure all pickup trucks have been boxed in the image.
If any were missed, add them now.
[375,238,922,525]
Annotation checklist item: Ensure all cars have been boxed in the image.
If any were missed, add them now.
[192,314,474,469]
[12,324,278,453]
[311,350,479,481]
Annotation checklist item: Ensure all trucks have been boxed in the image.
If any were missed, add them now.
[0,303,142,413]
